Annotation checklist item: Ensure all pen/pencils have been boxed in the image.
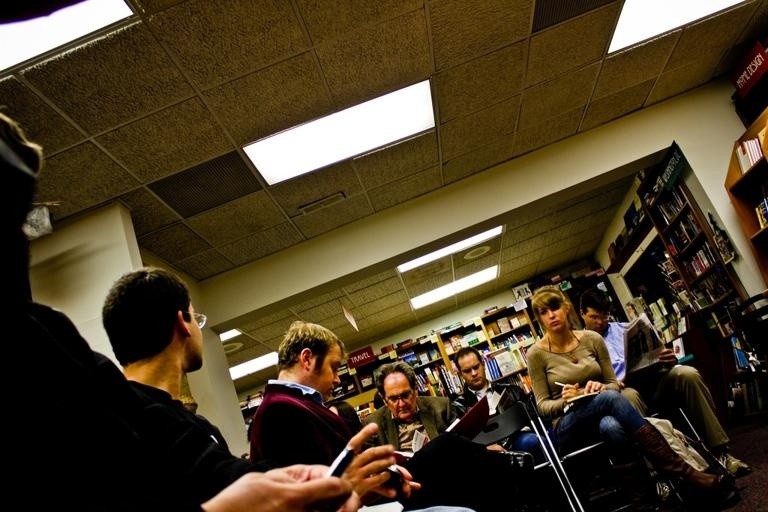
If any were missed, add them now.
[554,381,580,391]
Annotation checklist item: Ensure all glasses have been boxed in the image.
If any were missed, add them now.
[187,312,207,328]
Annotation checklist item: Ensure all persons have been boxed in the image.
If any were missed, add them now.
[1,103,753,511]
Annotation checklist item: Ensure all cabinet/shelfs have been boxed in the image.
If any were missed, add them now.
[229,104,768,431]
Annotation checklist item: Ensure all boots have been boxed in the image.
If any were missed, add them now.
[623,421,723,512]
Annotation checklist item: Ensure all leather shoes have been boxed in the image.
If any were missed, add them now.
[507,451,534,474]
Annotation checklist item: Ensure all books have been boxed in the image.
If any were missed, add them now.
[755,198,768,229]
[735,128,764,175]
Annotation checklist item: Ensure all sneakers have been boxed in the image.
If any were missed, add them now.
[722,454,751,478]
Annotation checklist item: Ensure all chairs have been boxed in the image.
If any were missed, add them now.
[470,390,686,510]
